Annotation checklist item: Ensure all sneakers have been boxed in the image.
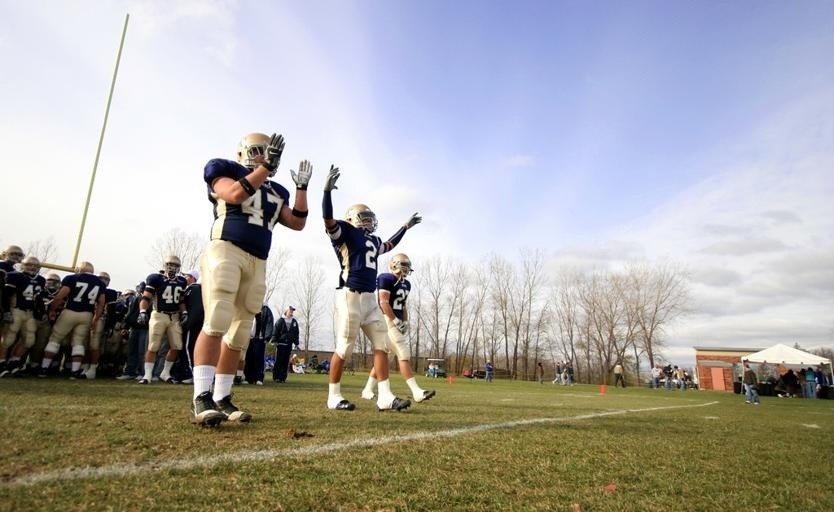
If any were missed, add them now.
[745,401,760,404]
[552,380,554,384]
[326,390,435,412]
[191,391,252,427]
[0,366,285,385]
[777,393,797,398]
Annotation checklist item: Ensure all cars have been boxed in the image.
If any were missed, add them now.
[464,369,486,379]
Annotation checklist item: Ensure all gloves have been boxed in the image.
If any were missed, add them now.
[405,212,423,229]
[394,317,408,333]
[262,132,285,171]
[323,163,340,191]
[290,160,313,190]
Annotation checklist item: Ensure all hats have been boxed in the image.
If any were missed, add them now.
[285,306,295,311]
[179,270,200,281]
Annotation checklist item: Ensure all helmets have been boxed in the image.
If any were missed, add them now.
[0,245,40,276]
[164,255,181,275]
[388,254,414,276]
[116,281,145,302]
[73,261,110,287]
[344,204,378,233]
[237,133,280,178]
[46,273,61,290]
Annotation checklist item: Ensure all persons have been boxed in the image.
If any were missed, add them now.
[537,362,543,384]
[0,244,300,387]
[485,359,493,382]
[649,363,692,392]
[428,363,435,374]
[612,361,625,388]
[774,366,826,399]
[551,360,574,385]
[742,365,761,406]
[185,130,313,428]
[356,253,437,404]
[320,165,422,412]
